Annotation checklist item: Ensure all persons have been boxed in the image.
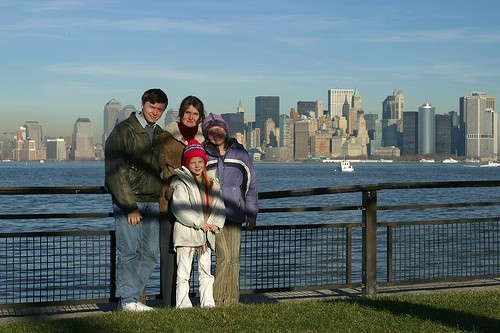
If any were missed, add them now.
[158,95,209,312]
[103,87,173,313]
[170,138,228,309]
[164,113,259,305]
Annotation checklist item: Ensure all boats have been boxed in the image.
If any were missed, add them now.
[480,161,500,168]
[442,157,458,163]
[418,158,435,163]
[333,162,355,173]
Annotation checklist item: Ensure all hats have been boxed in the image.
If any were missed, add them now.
[203,113,229,138]
[183,139,208,168]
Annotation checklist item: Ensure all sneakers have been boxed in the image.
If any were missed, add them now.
[121,302,153,312]
